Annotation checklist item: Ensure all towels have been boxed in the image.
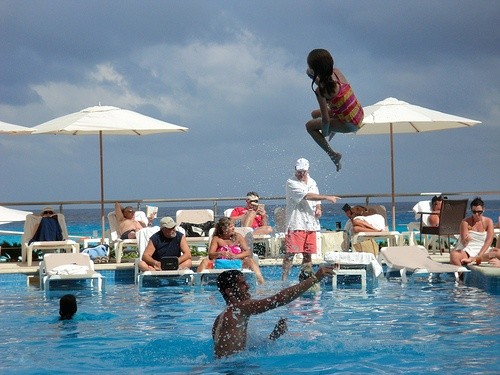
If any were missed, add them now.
[324,251,382,278]
[82,244,107,259]
[27,217,63,246]
[453,230,494,257]
[135,225,185,262]
[48,265,89,276]
[344,213,385,236]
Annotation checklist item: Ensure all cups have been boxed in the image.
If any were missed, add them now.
[93,230,98,238]
[235,220,241,227]
[336,222,341,231]
[476,256,481,266]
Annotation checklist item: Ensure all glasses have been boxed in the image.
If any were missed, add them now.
[129,210,135,213]
[169,225,176,229]
[471,210,484,214]
[44,210,54,214]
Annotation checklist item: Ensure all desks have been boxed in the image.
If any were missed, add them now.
[324,252,383,291]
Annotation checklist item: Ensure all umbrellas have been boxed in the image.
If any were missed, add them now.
[0,120,37,135]
[307,96,482,230]
[27,100,190,244]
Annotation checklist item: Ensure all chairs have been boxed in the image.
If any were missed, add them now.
[38,253,106,290]
[175,209,214,257]
[451,233,500,288]
[380,245,471,286]
[224,208,272,259]
[419,198,468,254]
[345,205,402,253]
[107,211,149,264]
[134,226,197,287]
[273,208,322,259]
[20,213,80,266]
[407,200,460,254]
[198,227,259,287]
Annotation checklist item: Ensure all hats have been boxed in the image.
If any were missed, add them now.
[295,158,309,171]
[246,192,259,201]
[42,207,54,215]
[160,217,177,229]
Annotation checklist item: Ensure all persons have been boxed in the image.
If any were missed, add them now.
[196,217,265,276]
[34,207,67,215]
[211,263,338,356]
[59,294,78,320]
[450,197,500,269]
[281,158,342,280]
[230,191,272,235]
[346,205,386,233]
[138,217,192,272]
[306,49,365,173]
[115,201,156,239]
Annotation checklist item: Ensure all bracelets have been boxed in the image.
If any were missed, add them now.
[310,272,318,283]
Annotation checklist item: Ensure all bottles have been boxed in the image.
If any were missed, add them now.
[488,237,497,259]
